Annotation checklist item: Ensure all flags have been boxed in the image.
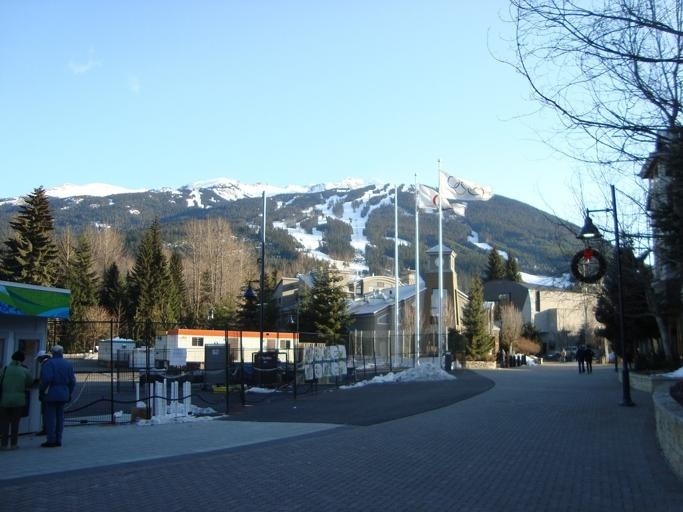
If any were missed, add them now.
[440,168,492,204]
[416,185,466,220]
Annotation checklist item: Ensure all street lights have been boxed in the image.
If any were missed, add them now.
[285,314,298,331]
[576,184,637,407]
[238,276,265,389]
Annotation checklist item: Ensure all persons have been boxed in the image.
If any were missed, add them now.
[34,348,50,438]
[559,348,566,363]
[40,345,77,449]
[0,350,33,451]
[574,345,585,374]
[583,344,595,374]
[496,347,519,369]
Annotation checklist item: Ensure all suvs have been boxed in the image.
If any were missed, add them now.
[544,344,579,361]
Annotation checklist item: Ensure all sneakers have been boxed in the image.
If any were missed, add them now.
[0,445,20,450]
[41,441,62,447]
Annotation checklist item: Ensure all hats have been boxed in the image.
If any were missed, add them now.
[33,350,50,360]
